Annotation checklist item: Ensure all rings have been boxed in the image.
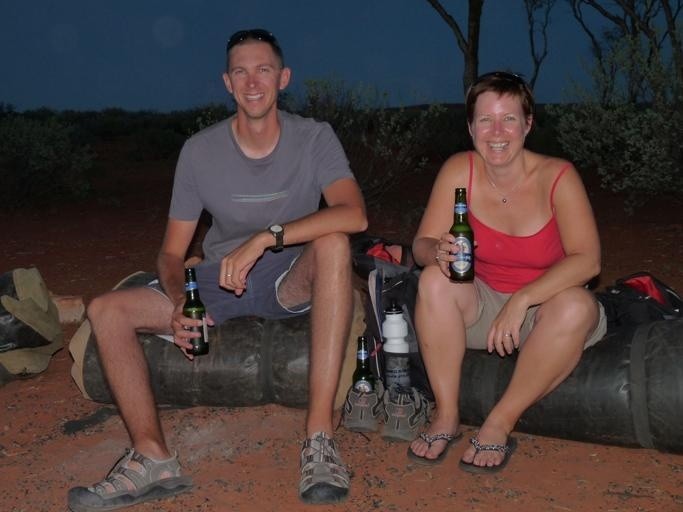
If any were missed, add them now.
[224,273,232,278]
[435,256,439,260]
[501,332,513,339]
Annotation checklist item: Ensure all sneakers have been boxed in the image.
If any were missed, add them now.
[338,373,388,436]
[379,382,432,445]
[296,430,352,505]
[63,447,193,511]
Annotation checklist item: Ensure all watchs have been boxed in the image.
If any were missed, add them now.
[265,223,286,254]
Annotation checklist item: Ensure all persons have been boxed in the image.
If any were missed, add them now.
[402,67,607,477]
[61,25,375,511]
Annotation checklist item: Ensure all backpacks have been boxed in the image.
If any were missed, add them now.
[593,270,683,324]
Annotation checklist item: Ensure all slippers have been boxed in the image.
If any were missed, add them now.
[456,432,520,478]
[403,425,465,467]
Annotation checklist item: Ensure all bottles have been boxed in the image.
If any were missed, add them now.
[381,299,409,355]
[446,187,475,283]
[183,266,210,357]
[352,336,374,393]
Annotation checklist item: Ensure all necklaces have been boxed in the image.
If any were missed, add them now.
[485,166,527,205]
